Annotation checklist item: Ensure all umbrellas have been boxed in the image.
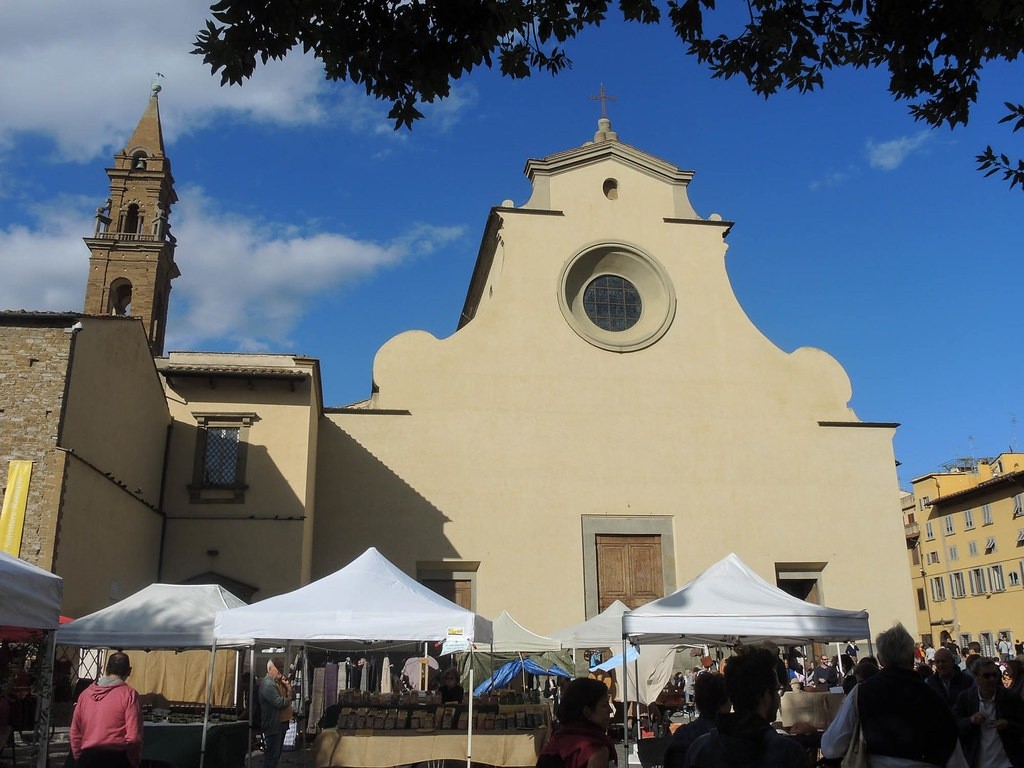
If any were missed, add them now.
[0,550,879,768]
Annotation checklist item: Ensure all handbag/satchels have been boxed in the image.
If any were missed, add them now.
[841,716,869,768]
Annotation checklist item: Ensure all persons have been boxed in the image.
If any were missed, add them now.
[69,651,144,768]
[666,625,1024,768]
[438,668,464,705]
[238,671,261,731]
[536,676,618,768]
[258,657,294,767]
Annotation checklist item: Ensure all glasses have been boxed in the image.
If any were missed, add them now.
[776,682,786,696]
[979,671,999,680]
[822,658,828,660]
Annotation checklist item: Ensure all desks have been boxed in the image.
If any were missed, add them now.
[142,720,250,768]
[313,725,548,768]
